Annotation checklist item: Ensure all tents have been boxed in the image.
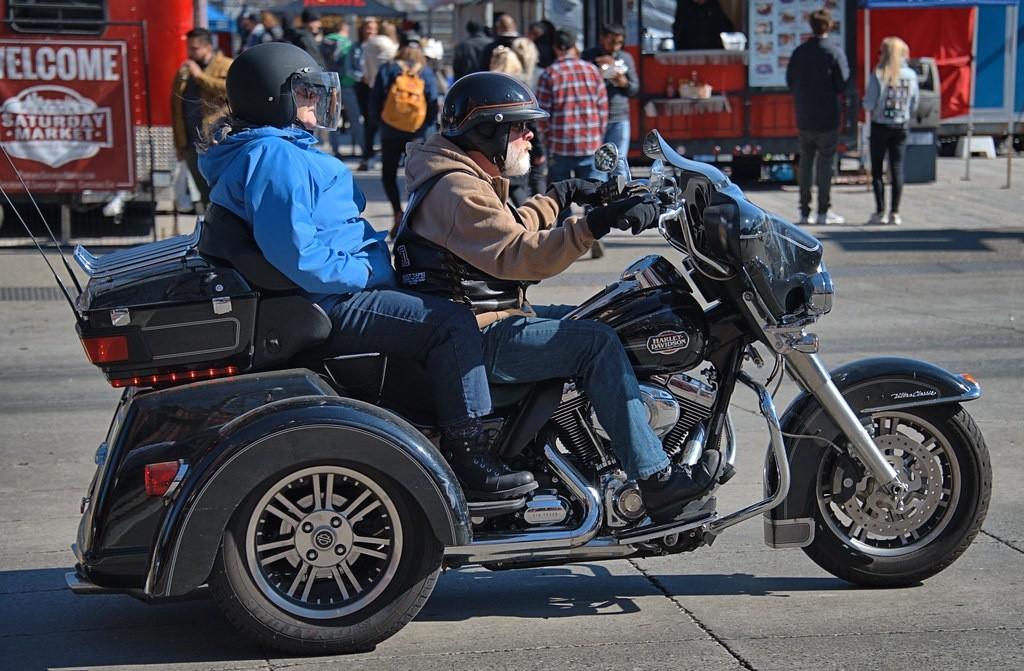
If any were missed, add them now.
[207,3,236,32]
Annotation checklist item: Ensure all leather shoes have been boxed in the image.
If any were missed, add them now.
[637,449,725,523]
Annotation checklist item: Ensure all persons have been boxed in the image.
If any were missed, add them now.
[862,37,920,226]
[423,37,454,139]
[257,9,307,52]
[534,27,609,260]
[580,23,640,182]
[785,9,851,223]
[490,45,547,206]
[344,16,380,171]
[319,15,382,159]
[391,70,726,524]
[483,14,523,73]
[372,39,438,240]
[298,8,328,71]
[237,12,258,55]
[454,20,495,81]
[529,21,557,68]
[170,27,234,214]
[173,42,538,502]
[512,37,546,96]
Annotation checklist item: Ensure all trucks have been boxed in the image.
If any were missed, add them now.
[450,1,1023,187]
[0,2,210,250]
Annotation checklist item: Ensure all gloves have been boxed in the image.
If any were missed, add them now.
[586,196,660,240]
[546,179,605,215]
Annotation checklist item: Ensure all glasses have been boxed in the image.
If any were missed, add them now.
[511,120,525,132]
[295,86,319,98]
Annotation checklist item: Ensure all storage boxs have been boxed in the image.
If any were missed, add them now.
[76,246,261,387]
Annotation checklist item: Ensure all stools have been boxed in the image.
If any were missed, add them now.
[955,135,996,160]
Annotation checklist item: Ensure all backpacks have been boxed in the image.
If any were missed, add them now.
[381,60,428,132]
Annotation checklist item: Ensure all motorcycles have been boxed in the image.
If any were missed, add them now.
[62,123,995,655]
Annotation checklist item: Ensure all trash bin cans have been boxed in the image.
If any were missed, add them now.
[904,128,936,184]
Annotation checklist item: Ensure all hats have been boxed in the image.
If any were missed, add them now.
[398,31,421,49]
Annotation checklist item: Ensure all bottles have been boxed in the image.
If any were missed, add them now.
[732,144,761,154]
[642,33,656,51]
[178,66,189,76]
[712,146,720,155]
[667,76,674,98]
[762,152,801,162]
[678,70,712,99]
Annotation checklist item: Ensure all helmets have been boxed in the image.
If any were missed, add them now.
[440,72,549,137]
[225,42,340,131]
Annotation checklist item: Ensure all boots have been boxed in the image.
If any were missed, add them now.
[440,427,540,501]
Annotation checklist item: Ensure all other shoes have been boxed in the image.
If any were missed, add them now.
[889,212,901,225]
[591,238,603,258]
[799,213,817,224]
[816,208,844,224]
[357,163,368,171]
[866,211,888,224]
